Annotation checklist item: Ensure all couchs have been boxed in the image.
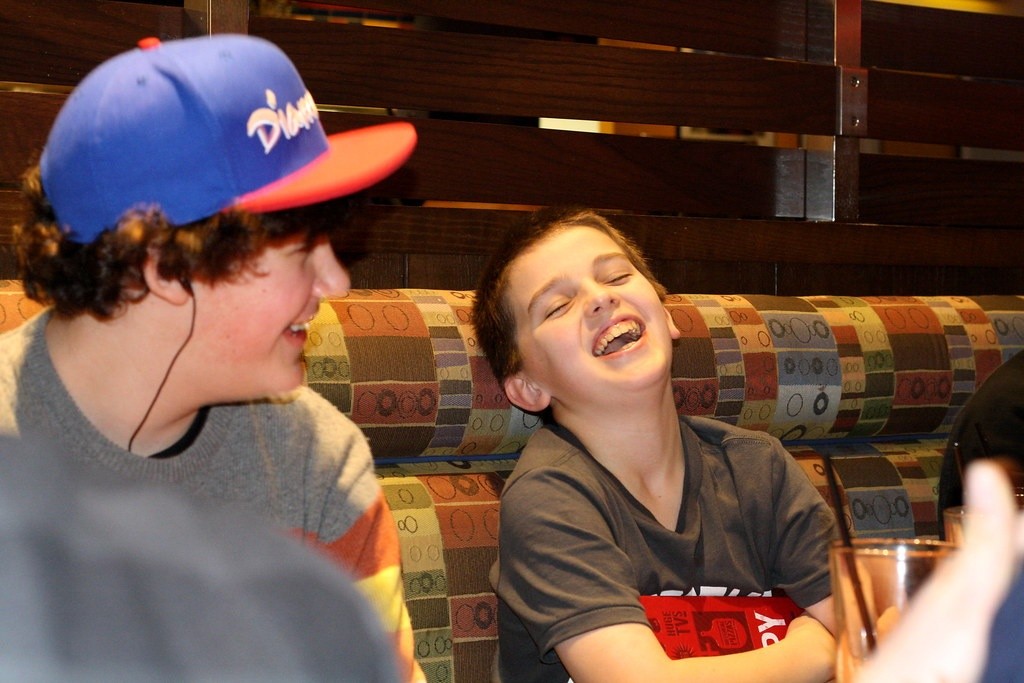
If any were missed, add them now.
[0,278,1024,683]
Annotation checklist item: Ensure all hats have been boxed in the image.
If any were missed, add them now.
[33,31,418,241]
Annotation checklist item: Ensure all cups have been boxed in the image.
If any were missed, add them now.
[943,505,964,543]
[829,538,958,683]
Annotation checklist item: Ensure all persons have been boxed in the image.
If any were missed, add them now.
[471,202,874,683]
[0,33,428,683]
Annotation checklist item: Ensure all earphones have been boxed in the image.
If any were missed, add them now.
[173,265,194,300]
